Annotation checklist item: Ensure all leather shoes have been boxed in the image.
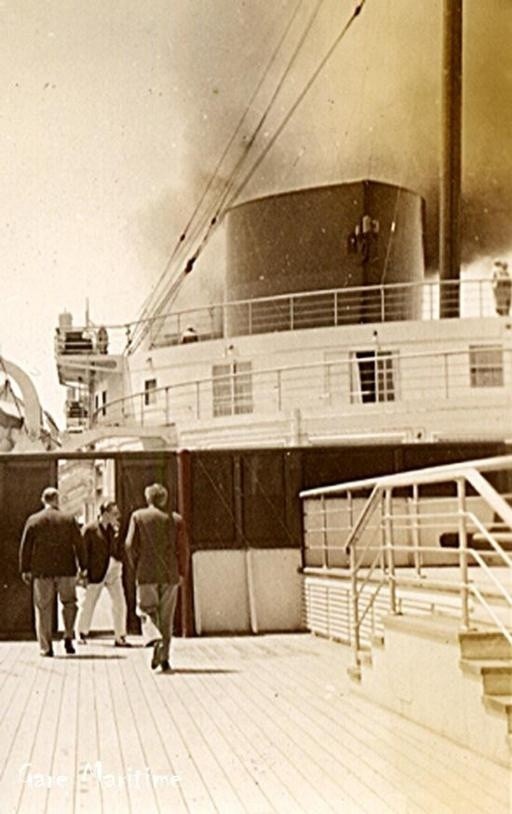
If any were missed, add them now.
[74,631,85,645]
[64,637,75,654]
[113,637,132,647]
[150,642,172,674]
[40,650,54,658]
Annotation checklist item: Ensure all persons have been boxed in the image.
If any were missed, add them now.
[72,498,132,647]
[502,262,509,279]
[180,327,199,343]
[123,478,192,673]
[491,258,510,317]
[18,484,91,658]
